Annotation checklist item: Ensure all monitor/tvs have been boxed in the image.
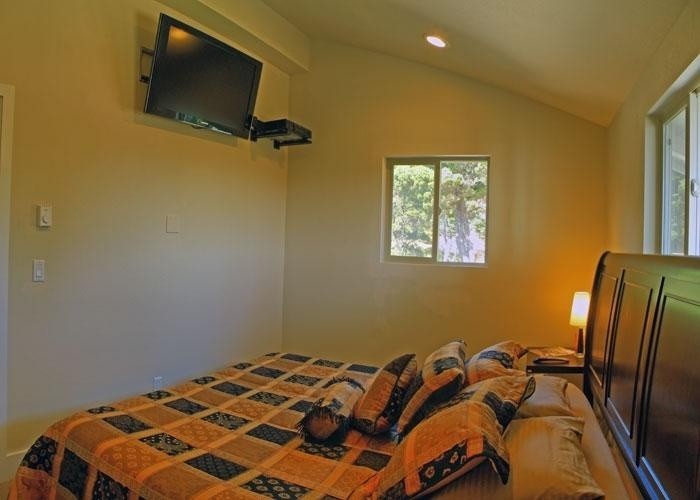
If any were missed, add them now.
[142,12,264,141]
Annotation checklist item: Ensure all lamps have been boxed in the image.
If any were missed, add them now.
[568,290,590,358]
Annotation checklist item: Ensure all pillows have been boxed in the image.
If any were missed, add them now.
[428,415,604,499]
[294,374,366,448]
[511,368,577,418]
[349,352,419,437]
[465,340,528,387]
[395,336,467,437]
[346,375,511,499]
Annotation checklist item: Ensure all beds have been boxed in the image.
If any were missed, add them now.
[8,250,700,499]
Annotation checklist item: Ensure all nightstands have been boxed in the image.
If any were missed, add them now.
[525,344,584,391]
